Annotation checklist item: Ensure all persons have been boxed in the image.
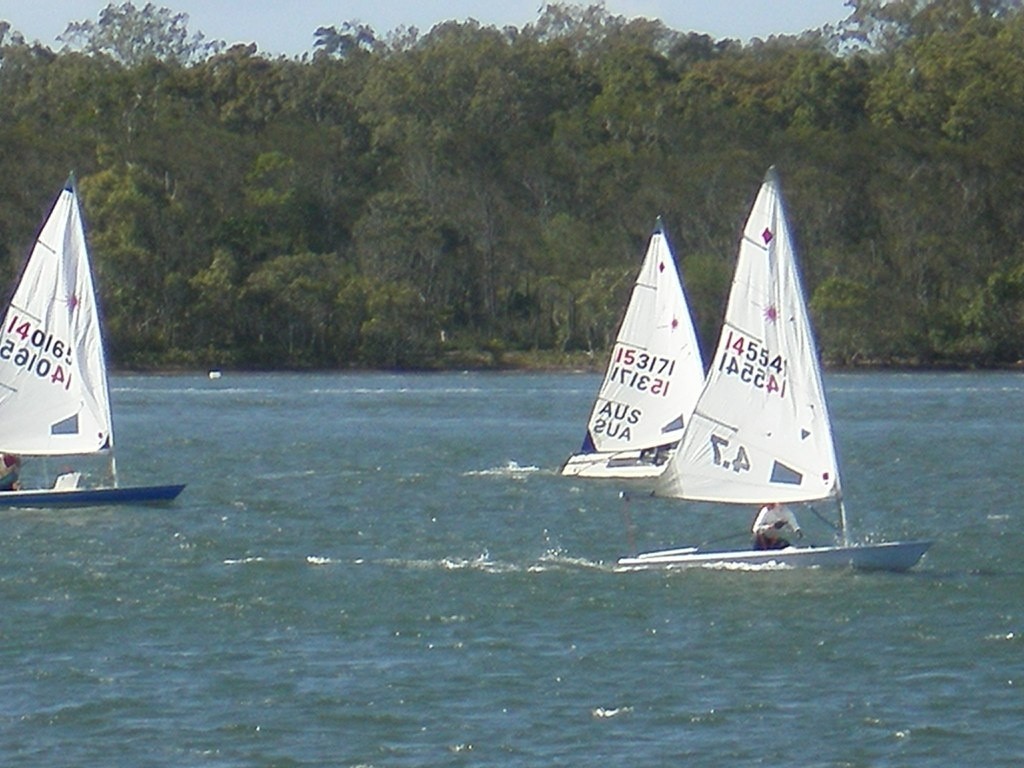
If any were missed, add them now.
[0,453,21,491]
[752,504,804,550]
[636,443,672,466]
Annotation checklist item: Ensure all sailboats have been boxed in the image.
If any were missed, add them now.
[0,171,187,508]
[614,165,934,570]
[559,215,707,478]
